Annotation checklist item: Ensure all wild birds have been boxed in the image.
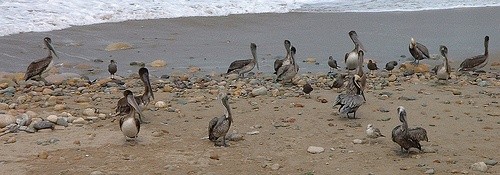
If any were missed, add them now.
[383,60,398,76]
[273,39,300,88]
[207,93,233,147]
[113,60,155,120]
[431,45,452,82]
[366,59,380,75]
[389,104,429,154]
[456,36,490,70]
[302,83,314,98]
[365,123,387,145]
[24,37,61,82]
[408,37,430,66]
[225,42,259,77]
[327,55,341,74]
[119,88,142,141]
[332,30,368,119]
[107,59,118,79]
